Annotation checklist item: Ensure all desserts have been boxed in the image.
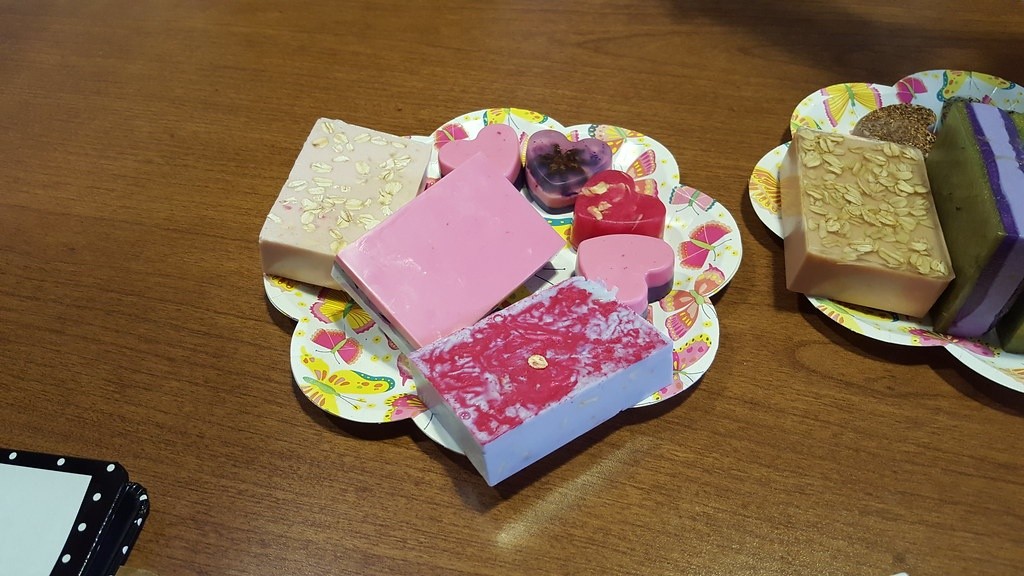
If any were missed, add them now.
[780,126,957,319]
[259,116,432,293]
[853,104,938,159]
[408,276,672,487]
[438,123,674,319]
[925,100,1024,355]
[331,149,569,358]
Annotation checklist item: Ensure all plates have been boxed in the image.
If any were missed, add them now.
[748,69,1022,397]
[260,108,742,453]
